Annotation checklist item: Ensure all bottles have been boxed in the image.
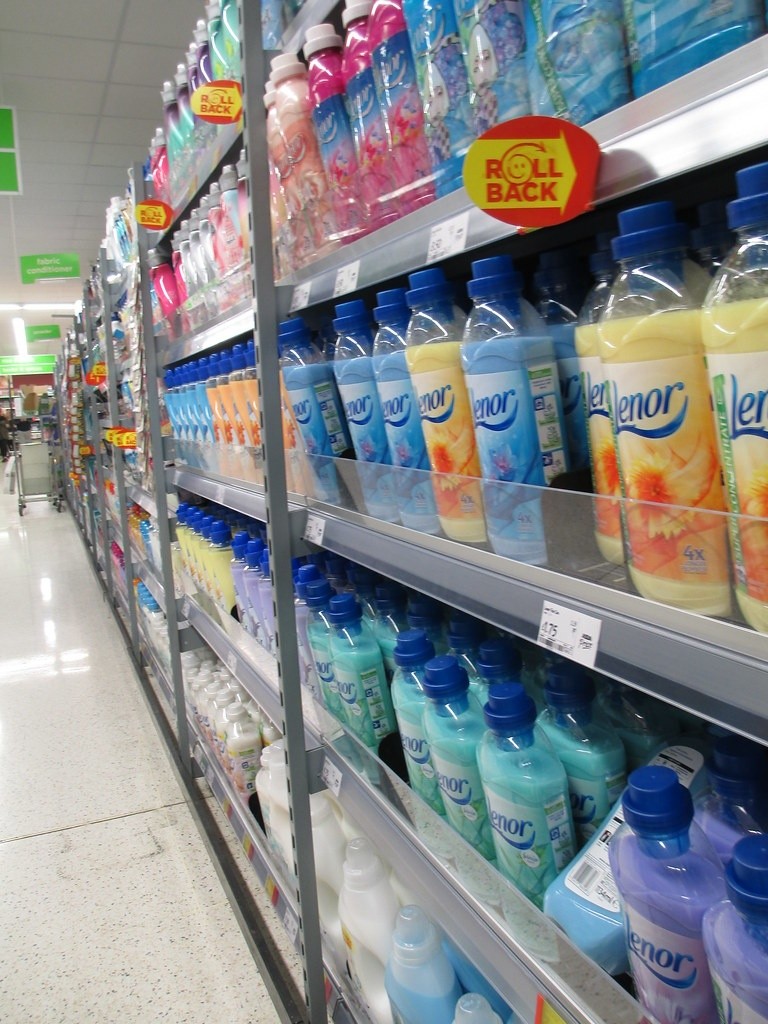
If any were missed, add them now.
[127,0,768,1024]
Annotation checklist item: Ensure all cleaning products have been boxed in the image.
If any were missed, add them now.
[103,0,768,1023]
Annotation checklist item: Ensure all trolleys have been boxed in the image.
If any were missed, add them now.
[14,443,65,516]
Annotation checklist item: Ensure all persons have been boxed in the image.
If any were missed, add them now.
[0,409,12,463]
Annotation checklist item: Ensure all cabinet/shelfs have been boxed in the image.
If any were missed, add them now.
[50,0,768,1024]
